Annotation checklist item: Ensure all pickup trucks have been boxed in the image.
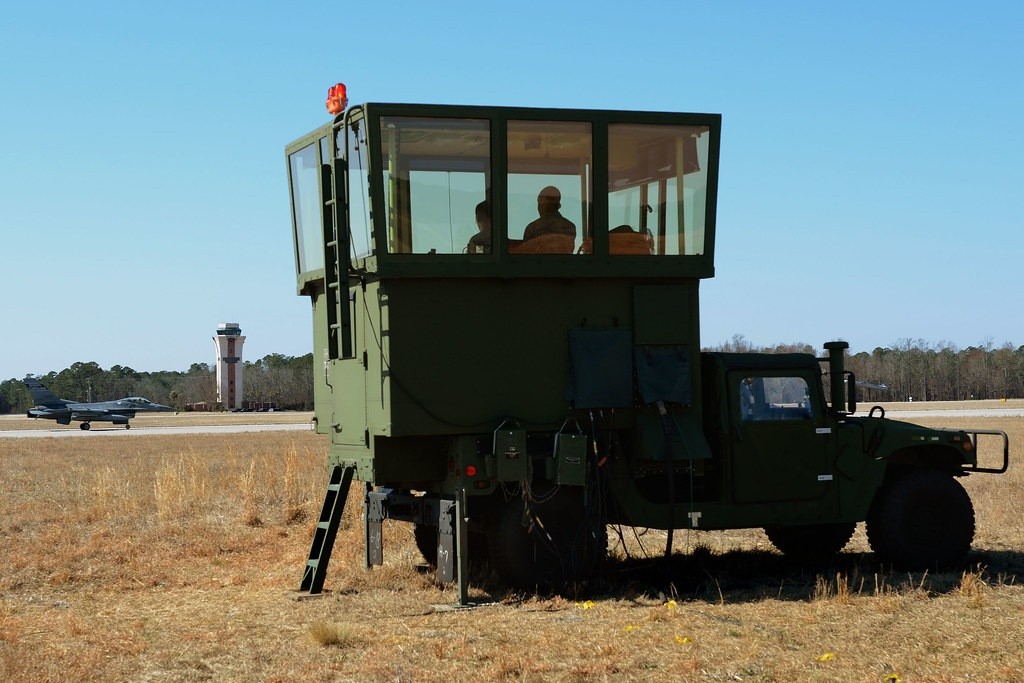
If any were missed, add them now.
[283,85,1012,596]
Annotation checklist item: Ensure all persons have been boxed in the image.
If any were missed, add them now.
[467,199,491,254]
[523,186,576,238]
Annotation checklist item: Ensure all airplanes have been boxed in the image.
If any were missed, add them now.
[23,377,178,430]
